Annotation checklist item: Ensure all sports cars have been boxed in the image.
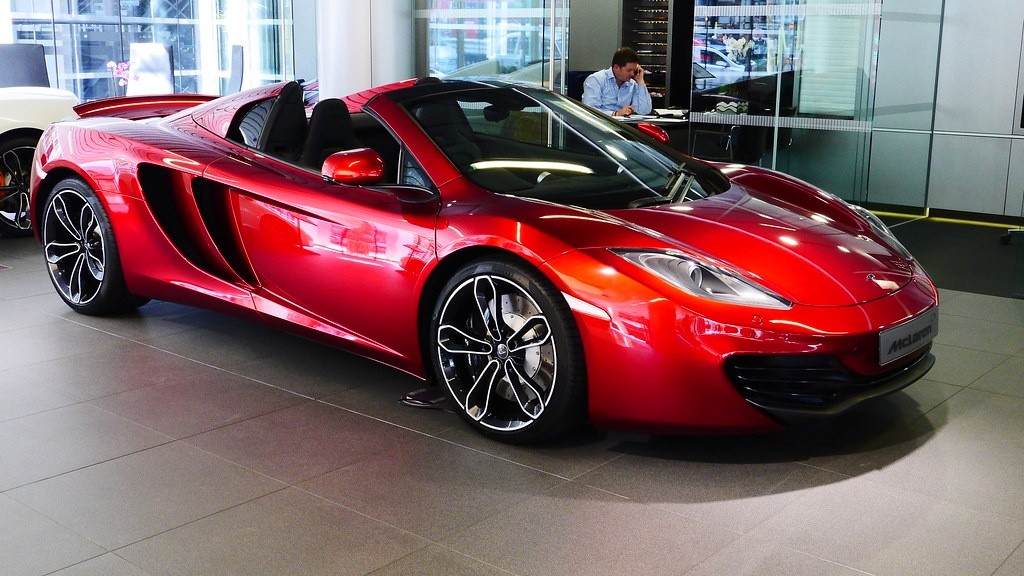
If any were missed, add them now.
[28,77,941,443]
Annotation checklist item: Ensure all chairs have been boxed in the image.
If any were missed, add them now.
[297,97,358,174]
[689,106,797,175]
[553,68,599,101]
[409,77,484,160]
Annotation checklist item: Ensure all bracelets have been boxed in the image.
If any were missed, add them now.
[615,111,617,116]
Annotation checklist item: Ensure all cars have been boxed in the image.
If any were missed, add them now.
[692,44,793,88]
[0,84,76,240]
[495,32,568,63]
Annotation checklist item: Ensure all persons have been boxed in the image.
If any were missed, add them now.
[581,48,653,116]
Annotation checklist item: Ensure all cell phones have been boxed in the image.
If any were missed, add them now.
[634,70,640,76]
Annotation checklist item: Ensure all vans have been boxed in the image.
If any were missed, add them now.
[427,29,459,72]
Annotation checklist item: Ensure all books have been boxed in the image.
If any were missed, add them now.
[610,108,688,122]
